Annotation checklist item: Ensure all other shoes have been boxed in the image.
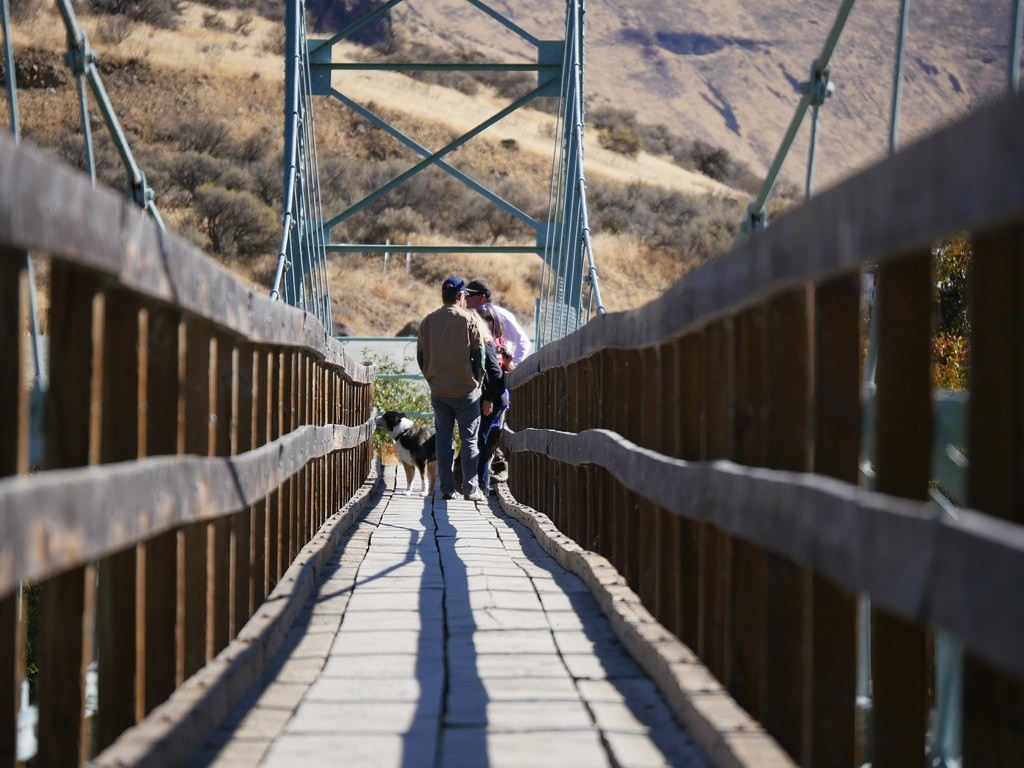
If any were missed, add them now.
[440,491,457,500]
[464,486,483,500]
[480,486,490,497]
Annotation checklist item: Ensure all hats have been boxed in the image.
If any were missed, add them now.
[465,279,491,298]
[442,276,466,291]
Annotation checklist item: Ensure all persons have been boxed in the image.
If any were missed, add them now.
[475,303,516,497]
[453,278,532,496]
[416,275,488,501]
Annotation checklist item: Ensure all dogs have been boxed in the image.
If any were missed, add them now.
[374,412,457,499]
[454,443,503,495]
